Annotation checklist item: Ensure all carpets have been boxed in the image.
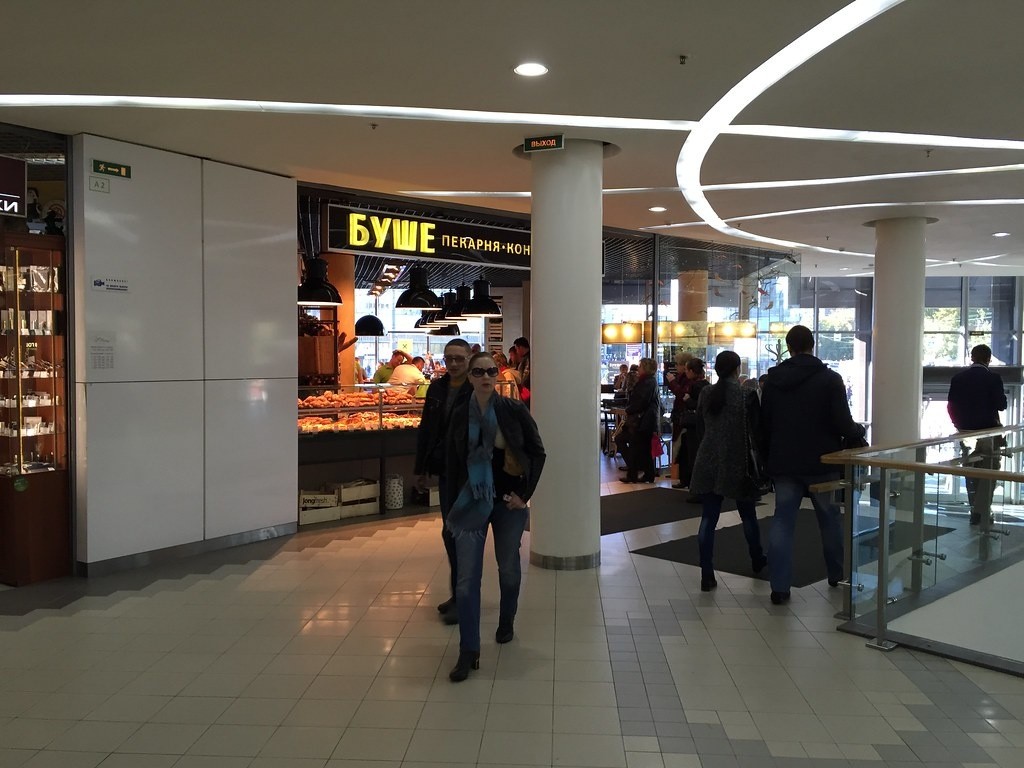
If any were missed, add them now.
[524,486,769,537]
[629,508,956,588]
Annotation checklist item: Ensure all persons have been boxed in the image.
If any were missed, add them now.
[945,344,1007,526]
[739,373,767,406]
[449,353,546,681]
[667,351,712,488]
[614,358,659,484]
[412,339,471,625]
[386,357,426,393]
[471,336,530,386]
[760,325,865,605]
[690,350,767,592]
[423,354,446,369]
[373,350,413,383]
[354,357,367,391]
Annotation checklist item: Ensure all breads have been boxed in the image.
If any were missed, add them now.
[303,329,358,353]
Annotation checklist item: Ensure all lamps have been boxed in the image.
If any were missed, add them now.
[297,254,342,306]
[355,315,388,336]
[394,261,503,335]
[602,255,788,346]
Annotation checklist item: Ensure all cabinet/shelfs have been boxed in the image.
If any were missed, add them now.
[0,230,72,588]
[297,382,430,444]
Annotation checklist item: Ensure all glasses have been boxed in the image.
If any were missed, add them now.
[443,357,468,363]
[469,367,498,378]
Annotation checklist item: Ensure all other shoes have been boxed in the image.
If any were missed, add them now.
[829,577,843,586]
[673,483,689,489]
[753,556,767,572]
[619,478,636,483]
[687,495,703,504]
[701,573,717,590]
[445,612,458,624]
[772,590,791,604]
[619,467,629,471]
[438,597,455,612]
[638,474,654,483]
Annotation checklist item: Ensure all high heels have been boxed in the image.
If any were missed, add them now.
[496,612,515,643]
[449,651,480,681]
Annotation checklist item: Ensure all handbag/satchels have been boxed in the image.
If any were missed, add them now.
[748,441,769,485]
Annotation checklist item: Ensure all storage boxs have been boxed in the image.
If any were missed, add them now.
[298,476,380,527]
[423,485,441,507]
